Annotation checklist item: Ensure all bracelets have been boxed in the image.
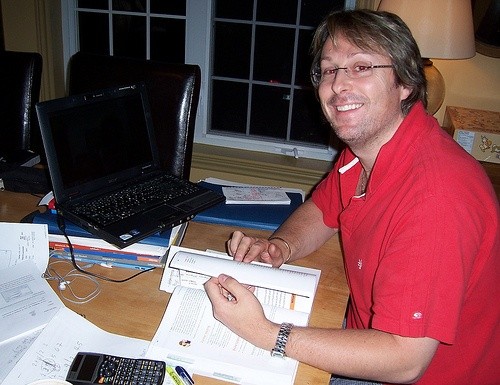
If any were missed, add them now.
[268,235,293,265]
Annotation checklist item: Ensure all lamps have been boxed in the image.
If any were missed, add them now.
[373,0,476,120]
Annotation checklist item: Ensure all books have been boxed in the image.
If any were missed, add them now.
[193,180,304,231]
[28,189,189,274]
[143,246,322,385]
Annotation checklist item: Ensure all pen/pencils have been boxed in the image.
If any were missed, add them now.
[167,365,195,385]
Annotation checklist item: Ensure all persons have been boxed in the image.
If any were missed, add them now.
[202,8,500,385]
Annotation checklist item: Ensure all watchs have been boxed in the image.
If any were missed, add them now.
[269,321,295,359]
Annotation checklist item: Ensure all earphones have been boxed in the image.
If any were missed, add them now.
[59,280,70,291]
[100,261,113,269]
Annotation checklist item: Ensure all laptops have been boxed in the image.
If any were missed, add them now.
[35,81,226,249]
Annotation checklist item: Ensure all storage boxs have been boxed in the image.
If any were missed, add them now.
[440,105,499,201]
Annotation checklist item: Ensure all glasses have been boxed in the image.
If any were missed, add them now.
[309,60,395,84]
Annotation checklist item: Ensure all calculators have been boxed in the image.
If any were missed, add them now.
[66,352,166,385]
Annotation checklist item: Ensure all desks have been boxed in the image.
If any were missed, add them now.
[0,154,353,384]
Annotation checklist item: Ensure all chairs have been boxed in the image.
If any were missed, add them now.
[65,50,202,189]
[0,50,45,159]
